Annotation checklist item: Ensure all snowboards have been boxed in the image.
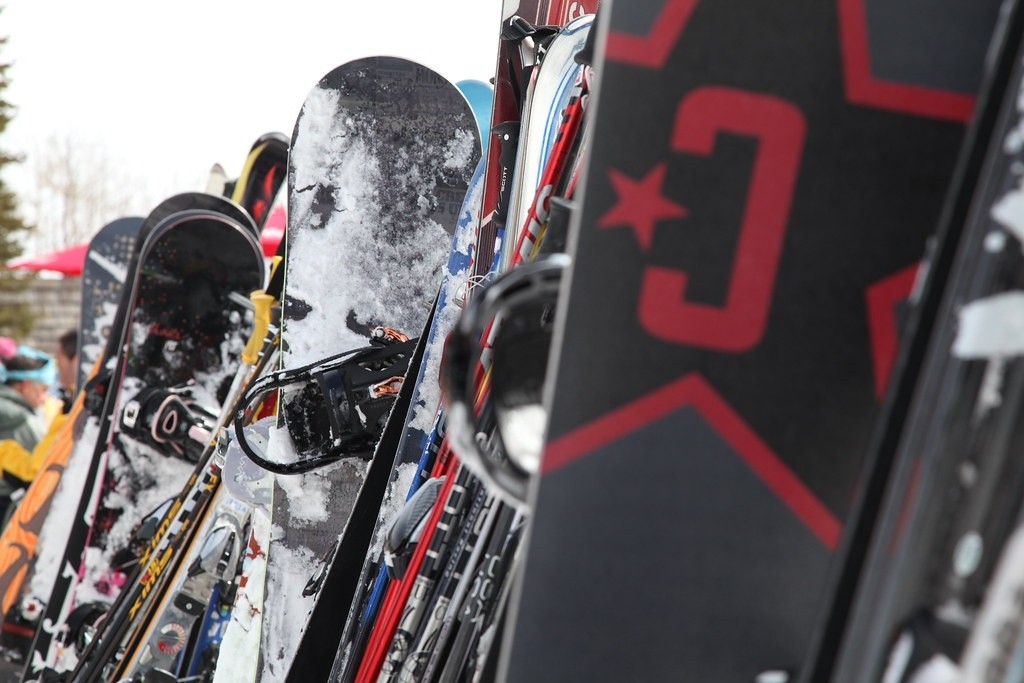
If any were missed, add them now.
[0,0,1023,683]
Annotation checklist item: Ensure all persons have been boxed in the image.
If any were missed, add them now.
[0,328,78,539]
